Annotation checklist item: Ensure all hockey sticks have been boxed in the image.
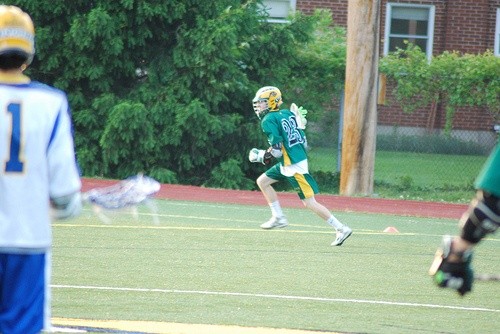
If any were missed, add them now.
[77,174,161,210]
[289,103,308,130]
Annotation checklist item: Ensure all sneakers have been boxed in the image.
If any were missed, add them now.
[261,215,289,229]
[331,225,352,246]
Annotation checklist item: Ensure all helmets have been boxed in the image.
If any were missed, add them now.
[252,85,283,119]
[0,5,36,69]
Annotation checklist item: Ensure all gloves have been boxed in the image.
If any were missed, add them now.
[429,235,474,297]
[248,148,271,167]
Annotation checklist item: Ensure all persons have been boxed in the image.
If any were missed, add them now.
[429,138,500,298]
[0,4,83,334]
[248,85,353,248]
